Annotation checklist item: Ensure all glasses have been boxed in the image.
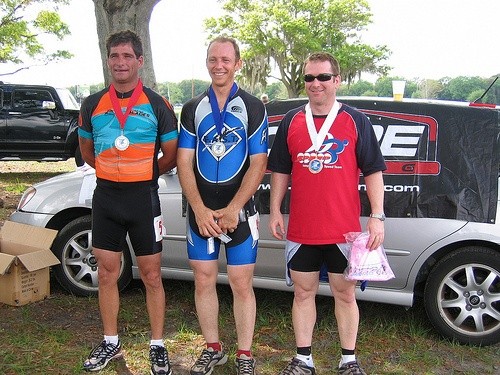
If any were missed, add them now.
[303,73,336,82]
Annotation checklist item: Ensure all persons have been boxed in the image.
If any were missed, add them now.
[265,52,388,375]
[77,29,179,375]
[176,36,269,375]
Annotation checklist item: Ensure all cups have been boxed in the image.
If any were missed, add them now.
[392,81,406,100]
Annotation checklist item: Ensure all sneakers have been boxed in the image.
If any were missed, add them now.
[233,353,256,375]
[148,345,172,375]
[83,337,122,371]
[189,343,228,375]
[279,357,317,375]
[337,358,367,375]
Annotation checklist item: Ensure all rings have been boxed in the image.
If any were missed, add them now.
[379,239,382,242]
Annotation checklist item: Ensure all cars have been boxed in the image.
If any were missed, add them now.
[0,82,86,168]
[8,97,500,346]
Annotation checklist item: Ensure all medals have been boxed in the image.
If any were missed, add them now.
[114,135,130,152]
[308,159,323,174]
[211,141,225,156]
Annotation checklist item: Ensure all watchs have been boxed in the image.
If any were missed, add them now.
[370,213,386,222]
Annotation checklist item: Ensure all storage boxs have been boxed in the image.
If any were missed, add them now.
[0,219,61,307]
